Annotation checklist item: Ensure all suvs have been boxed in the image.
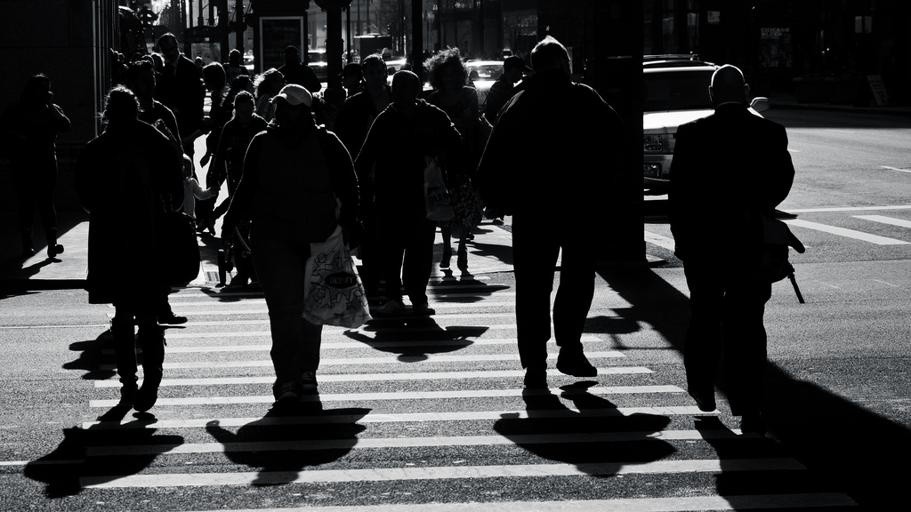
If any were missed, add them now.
[640,52,771,195]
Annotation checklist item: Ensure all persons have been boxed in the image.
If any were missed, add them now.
[116,56,198,325]
[353,69,473,316]
[76,83,185,415]
[222,84,357,414]
[670,64,794,412]
[112,35,522,287]
[484,36,624,389]
[2,73,71,279]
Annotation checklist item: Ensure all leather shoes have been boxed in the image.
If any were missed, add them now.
[158,315,187,324]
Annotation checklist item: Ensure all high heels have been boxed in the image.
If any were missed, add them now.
[440,249,452,266]
[458,244,466,254]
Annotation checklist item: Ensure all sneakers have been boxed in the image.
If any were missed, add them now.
[267,392,299,418]
[121,386,138,405]
[556,345,597,375]
[48,245,64,257]
[301,394,322,414]
[386,302,409,313]
[413,302,435,312]
[524,371,546,387]
[136,384,156,410]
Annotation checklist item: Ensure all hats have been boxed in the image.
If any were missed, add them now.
[269,83,312,108]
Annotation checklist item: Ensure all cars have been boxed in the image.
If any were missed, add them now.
[463,58,522,110]
[309,46,328,80]
